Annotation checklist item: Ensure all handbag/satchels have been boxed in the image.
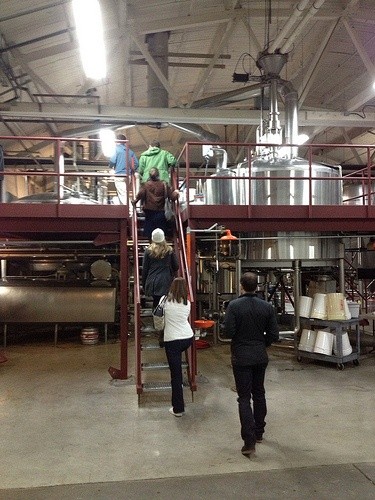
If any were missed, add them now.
[152,296,169,330]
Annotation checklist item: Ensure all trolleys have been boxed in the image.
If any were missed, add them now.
[287,315,364,371]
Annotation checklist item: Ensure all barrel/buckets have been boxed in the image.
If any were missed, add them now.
[344,298,351,320]
[311,293,327,319]
[332,330,353,356]
[298,329,317,352]
[327,293,344,320]
[300,296,313,318]
[313,331,334,355]
[348,303,361,317]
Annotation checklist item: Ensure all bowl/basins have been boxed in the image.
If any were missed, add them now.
[194,320,214,327]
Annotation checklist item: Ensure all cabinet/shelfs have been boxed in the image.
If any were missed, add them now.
[292,315,360,364]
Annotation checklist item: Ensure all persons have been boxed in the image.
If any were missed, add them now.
[158,278,194,416]
[142,227,179,347]
[222,272,280,453]
[109,134,138,216]
[137,139,176,184]
[131,167,178,240]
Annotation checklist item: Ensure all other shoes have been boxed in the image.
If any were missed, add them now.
[256,435,262,442]
[170,407,182,416]
[241,443,256,454]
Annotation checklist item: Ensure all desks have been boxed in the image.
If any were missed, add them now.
[363,312,375,354]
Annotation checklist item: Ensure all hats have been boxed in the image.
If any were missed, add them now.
[151,228,165,243]
